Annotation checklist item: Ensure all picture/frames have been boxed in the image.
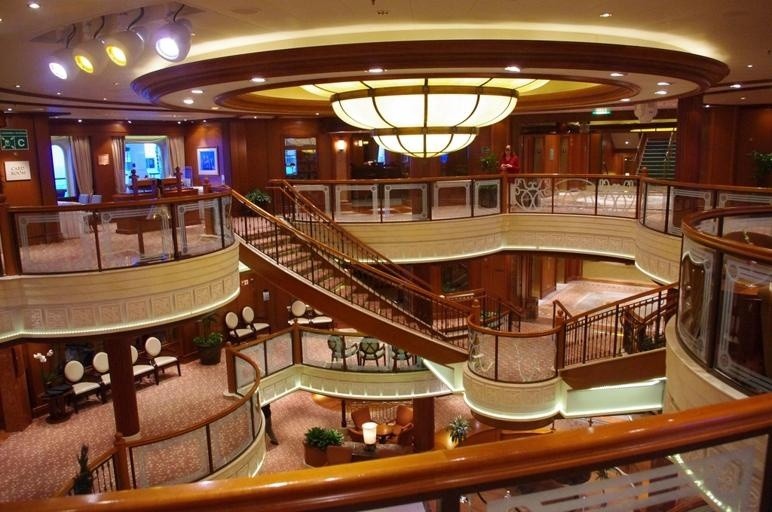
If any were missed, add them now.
[198,146,220,176]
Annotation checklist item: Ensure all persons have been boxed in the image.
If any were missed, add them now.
[261,404,278,446]
[499,145,520,207]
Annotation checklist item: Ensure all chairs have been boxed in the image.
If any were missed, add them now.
[63,336,182,415]
[225,306,272,346]
[328,335,413,369]
[288,300,335,333]
[348,404,414,445]
[303,441,401,469]
[77,194,103,234]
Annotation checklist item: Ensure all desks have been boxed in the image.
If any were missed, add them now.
[58,201,84,239]
[41,384,73,419]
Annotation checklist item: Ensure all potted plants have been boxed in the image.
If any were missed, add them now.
[245,187,272,218]
[747,149,772,185]
[191,312,225,365]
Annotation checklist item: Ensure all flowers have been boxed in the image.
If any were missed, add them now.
[33,348,55,369]
[443,414,475,446]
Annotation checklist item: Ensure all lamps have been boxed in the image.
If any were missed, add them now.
[361,421,378,451]
[47,3,193,79]
[300,76,549,159]
